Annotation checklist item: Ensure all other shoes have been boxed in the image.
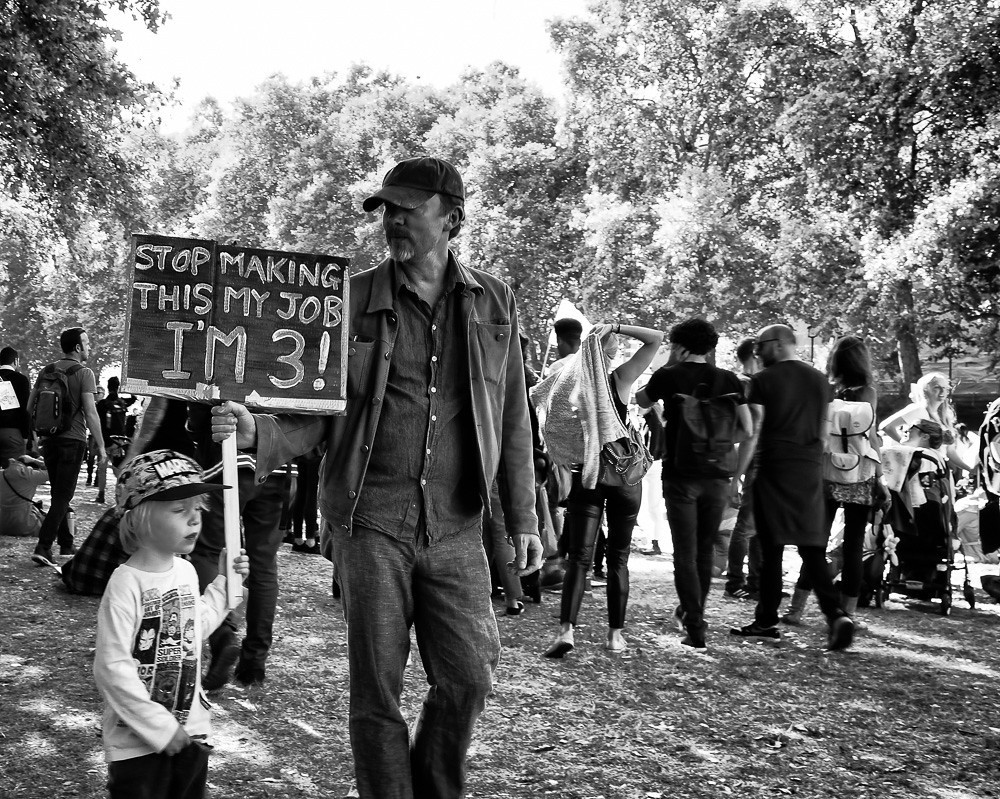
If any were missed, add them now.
[730,623,781,642]
[606,635,627,653]
[60,543,77,558]
[680,636,707,651]
[508,600,526,618]
[95,491,105,503]
[829,618,853,648]
[203,633,269,689]
[545,632,574,658]
[31,543,58,567]
[85,474,100,487]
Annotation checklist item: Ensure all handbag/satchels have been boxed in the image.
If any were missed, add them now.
[599,421,654,488]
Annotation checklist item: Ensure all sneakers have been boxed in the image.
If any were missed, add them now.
[724,583,754,599]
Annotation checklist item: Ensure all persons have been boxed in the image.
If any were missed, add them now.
[211,157,544,799]
[0,327,323,688]
[93,450,249,799]
[483,317,1000,658]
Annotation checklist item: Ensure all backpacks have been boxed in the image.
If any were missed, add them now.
[822,398,880,484]
[671,382,743,479]
[31,363,85,436]
[104,397,128,443]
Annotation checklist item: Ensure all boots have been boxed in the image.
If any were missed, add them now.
[778,588,811,624]
[840,593,868,631]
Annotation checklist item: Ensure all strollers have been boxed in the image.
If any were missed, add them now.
[872,445,977,616]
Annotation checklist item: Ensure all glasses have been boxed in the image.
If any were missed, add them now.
[905,423,927,436]
[757,338,781,351]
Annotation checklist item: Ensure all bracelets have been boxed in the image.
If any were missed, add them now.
[610,324,621,333]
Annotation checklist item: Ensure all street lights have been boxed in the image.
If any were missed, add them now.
[808,326,818,364]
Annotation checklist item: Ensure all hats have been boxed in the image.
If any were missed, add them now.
[362,156,464,213]
[115,449,233,514]
